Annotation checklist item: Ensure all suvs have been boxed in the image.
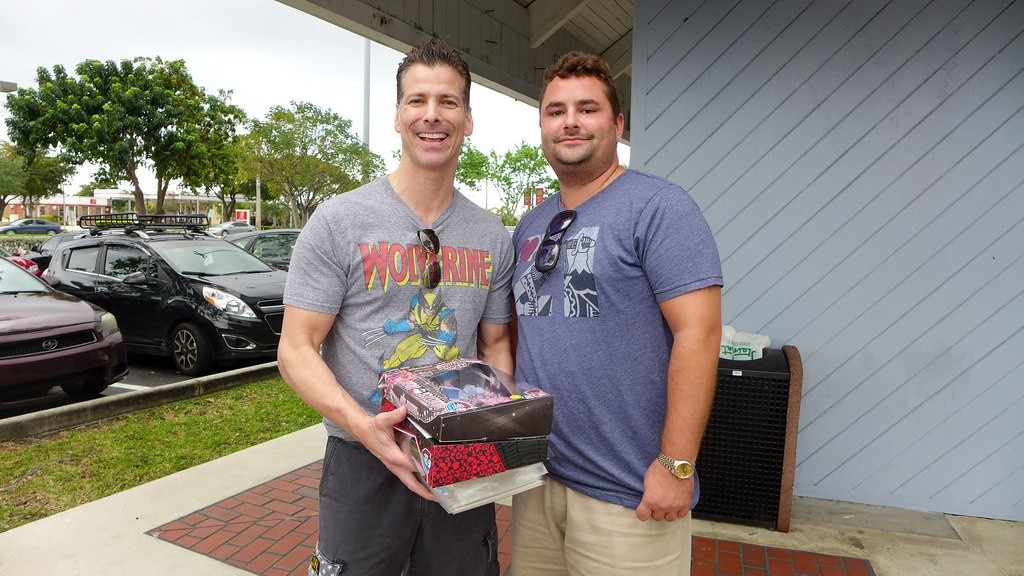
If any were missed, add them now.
[40,212,289,376]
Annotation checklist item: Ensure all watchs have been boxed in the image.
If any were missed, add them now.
[657,452,696,481]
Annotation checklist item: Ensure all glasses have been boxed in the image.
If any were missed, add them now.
[418,229,442,290]
[535,210,577,273]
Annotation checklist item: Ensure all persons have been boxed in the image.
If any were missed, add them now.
[511,50,723,573]
[273,36,518,574]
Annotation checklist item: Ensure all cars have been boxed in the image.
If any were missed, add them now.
[0,218,64,235]
[0,256,130,398]
[222,228,303,272]
[205,221,255,236]
[25,232,94,271]
[0,247,40,277]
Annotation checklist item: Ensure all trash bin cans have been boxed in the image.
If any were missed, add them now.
[691,343,805,532]
